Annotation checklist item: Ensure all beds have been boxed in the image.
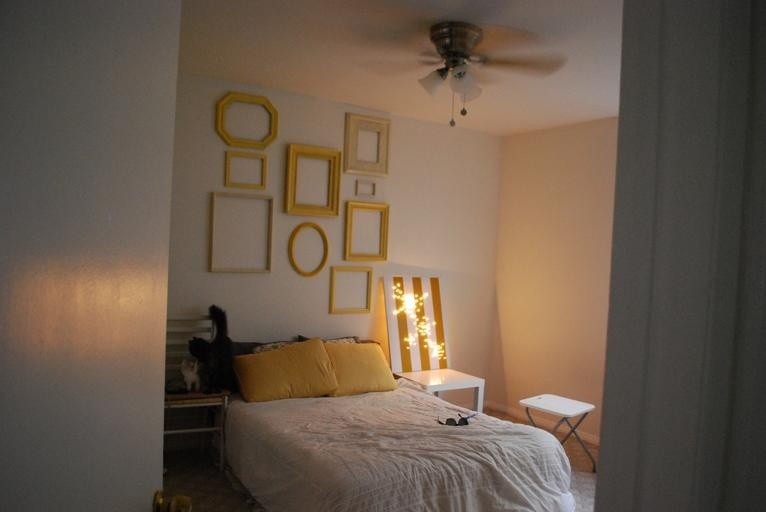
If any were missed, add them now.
[225,341,571,511]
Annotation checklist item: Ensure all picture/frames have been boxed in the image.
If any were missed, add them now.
[287,222,329,276]
[222,151,269,191]
[209,191,275,275]
[354,179,377,199]
[285,144,341,218]
[345,202,390,261]
[213,92,279,150]
[328,267,372,313]
[344,110,391,176]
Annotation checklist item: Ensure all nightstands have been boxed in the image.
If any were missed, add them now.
[394,368,483,415]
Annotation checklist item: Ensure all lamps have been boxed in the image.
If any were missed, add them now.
[358,22,560,126]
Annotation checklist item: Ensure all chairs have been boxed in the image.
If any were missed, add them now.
[163,319,228,471]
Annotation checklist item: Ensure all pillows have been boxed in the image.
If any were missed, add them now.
[322,343,396,397]
[231,337,338,402]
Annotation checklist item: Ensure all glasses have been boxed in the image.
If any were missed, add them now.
[436,413,469,426]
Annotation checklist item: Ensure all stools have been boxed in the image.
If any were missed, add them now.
[520,393,597,476]
